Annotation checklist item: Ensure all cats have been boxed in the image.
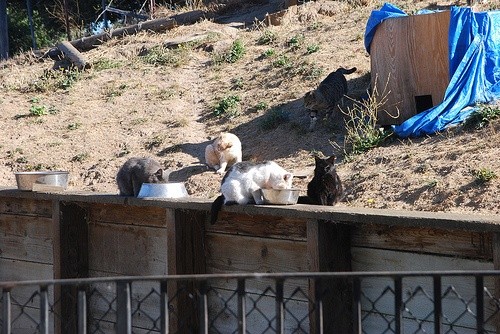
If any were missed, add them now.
[303,67,357,132]
[306,154,343,206]
[116,156,168,197]
[204,132,243,175]
[209,160,294,226]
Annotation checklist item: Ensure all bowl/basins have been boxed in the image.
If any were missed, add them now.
[261,188,300,205]
[15,171,68,191]
[136,182,189,200]
